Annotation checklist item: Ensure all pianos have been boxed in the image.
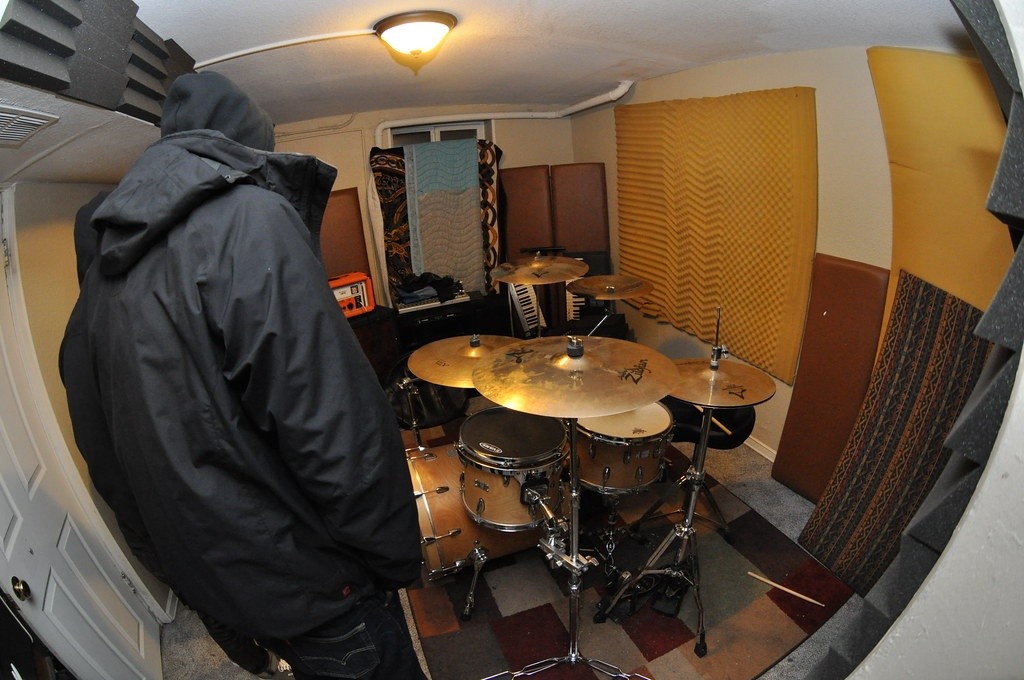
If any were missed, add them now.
[565,255,591,322]
[507,281,548,333]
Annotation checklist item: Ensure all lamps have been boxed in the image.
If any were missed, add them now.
[373,10,458,59]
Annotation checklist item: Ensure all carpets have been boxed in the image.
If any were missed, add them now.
[406,472,854,680]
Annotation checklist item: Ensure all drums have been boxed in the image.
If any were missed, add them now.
[456,405,568,534]
[568,401,676,498]
[404,441,548,582]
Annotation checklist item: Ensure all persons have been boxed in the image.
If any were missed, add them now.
[56,71,424,680]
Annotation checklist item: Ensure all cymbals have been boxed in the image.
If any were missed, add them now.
[407,334,525,389]
[489,255,590,286]
[665,357,777,411]
[565,274,655,301]
[471,335,682,419]
